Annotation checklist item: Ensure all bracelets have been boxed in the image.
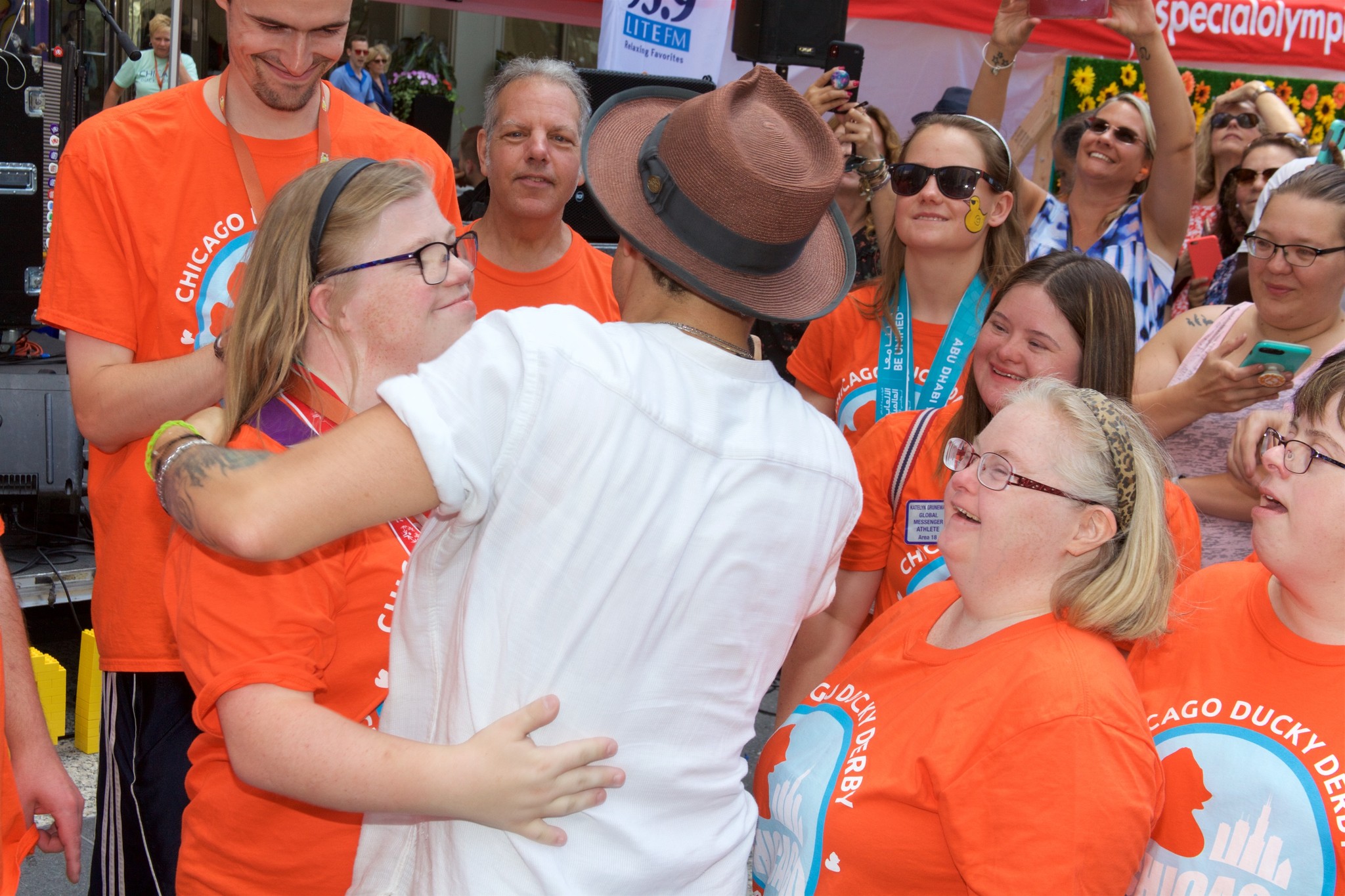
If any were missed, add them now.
[1250,86,1273,102]
[155,438,214,517]
[144,419,201,482]
[856,154,892,202]
[982,42,1017,76]
[1171,474,1186,485]
[155,433,207,480]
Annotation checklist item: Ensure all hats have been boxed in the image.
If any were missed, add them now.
[582,66,858,323]
[911,87,974,123]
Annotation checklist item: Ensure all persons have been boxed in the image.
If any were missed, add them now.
[746,65,906,388]
[1115,348,1345,896]
[144,64,865,896]
[461,55,622,326]
[775,113,1203,735]
[364,44,400,122]
[452,124,491,223]
[328,33,381,113]
[1128,163,1345,571]
[103,14,199,109]
[169,154,627,896]
[0,515,86,896]
[750,376,1178,896]
[964,1,1197,356]
[35,0,467,896]
[561,182,619,245]
[1166,79,1345,323]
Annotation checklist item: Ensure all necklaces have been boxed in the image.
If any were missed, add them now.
[218,61,330,224]
[652,321,755,360]
[155,53,169,91]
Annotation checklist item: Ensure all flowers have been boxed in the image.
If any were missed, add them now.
[387,32,467,134]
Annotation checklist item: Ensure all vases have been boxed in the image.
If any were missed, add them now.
[406,92,454,152]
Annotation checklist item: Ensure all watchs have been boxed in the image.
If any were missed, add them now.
[213,325,234,365]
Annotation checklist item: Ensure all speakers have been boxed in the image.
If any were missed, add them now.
[0,49,60,330]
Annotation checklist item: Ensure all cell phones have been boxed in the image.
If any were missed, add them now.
[1237,340,1311,388]
[1187,235,1223,288]
[822,40,865,115]
[1027,0,1109,20]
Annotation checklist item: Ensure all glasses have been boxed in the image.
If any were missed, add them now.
[1210,111,1261,130]
[1083,115,1154,156]
[354,48,370,56]
[374,59,388,63]
[308,230,481,286]
[1243,230,1345,268]
[1259,426,1345,475]
[943,437,1103,508]
[887,163,1004,199]
[1235,166,1283,188]
